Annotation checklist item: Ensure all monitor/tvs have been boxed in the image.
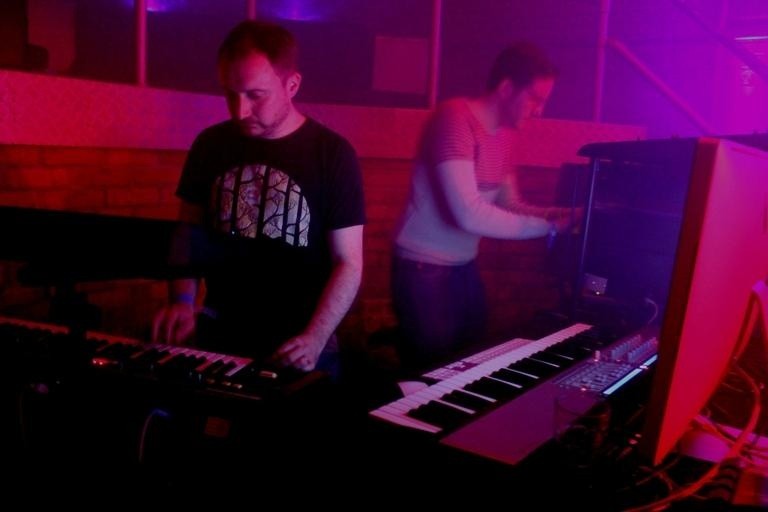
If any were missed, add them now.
[640,138,768,479]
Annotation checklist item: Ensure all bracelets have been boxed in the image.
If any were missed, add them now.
[172,293,195,302]
[550,222,557,241]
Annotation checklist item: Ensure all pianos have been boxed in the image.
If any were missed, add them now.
[0,317,307,420]
[369,323,664,466]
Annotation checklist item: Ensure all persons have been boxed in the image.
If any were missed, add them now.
[391,39,586,365]
[147,20,368,375]
